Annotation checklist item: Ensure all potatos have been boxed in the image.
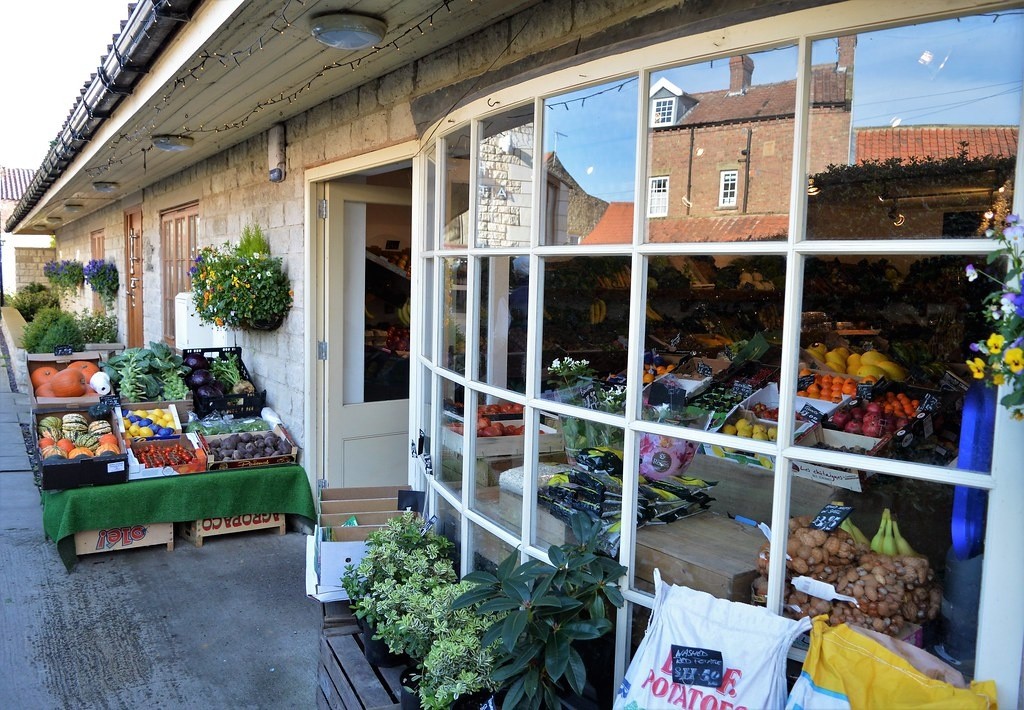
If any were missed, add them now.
[207,431,292,462]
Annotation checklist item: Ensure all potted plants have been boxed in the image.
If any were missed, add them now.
[397,582,511,710]
[339,508,457,668]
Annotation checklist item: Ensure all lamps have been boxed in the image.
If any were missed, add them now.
[310,15,388,50]
[32,226,47,230]
[45,217,62,224]
[150,135,194,153]
[63,206,84,213]
[92,183,121,193]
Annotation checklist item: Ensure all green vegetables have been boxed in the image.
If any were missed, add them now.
[185,421,272,436]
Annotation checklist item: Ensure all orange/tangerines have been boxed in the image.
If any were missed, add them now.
[643,363,676,383]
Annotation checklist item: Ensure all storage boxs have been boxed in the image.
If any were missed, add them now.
[442,421,565,487]
[24,352,114,409]
[123,433,207,480]
[823,381,947,456]
[182,346,267,420]
[779,366,868,404]
[319,527,424,593]
[622,342,730,454]
[317,512,423,563]
[702,408,811,473]
[120,385,194,423]
[738,383,835,423]
[181,424,302,547]
[70,486,175,558]
[718,362,780,393]
[319,485,413,501]
[115,404,181,441]
[792,429,882,492]
[319,498,414,515]
[31,406,128,491]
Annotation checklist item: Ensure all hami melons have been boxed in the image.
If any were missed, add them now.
[805,341,906,381]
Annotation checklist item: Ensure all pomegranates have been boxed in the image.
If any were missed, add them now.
[796,368,921,420]
[830,401,911,437]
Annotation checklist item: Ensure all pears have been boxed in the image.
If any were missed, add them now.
[720,418,777,442]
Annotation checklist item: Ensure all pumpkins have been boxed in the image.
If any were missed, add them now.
[36,404,120,464]
[31,360,102,397]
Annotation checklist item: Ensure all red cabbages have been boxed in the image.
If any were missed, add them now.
[182,353,226,398]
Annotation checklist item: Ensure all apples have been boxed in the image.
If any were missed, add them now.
[447,403,547,437]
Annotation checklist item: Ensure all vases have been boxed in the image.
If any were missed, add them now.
[565,441,622,467]
[240,316,284,332]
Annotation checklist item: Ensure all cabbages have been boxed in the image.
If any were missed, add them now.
[118,365,190,403]
[736,271,773,290]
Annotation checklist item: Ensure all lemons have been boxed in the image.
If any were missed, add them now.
[124,408,176,438]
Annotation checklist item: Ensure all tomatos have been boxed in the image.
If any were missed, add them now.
[133,443,199,468]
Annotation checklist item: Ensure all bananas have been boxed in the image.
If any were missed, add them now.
[647,300,664,321]
[588,297,606,325]
[830,501,918,557]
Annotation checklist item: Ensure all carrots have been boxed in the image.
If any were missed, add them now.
[594,271,630,288]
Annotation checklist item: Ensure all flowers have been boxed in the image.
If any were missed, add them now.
[83,258,119,306]
[43,259,59,285]
[185,226,290,333]
[547,356,626,450]
[58,259,85,298]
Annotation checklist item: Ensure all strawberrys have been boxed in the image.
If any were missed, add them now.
[748,403,804,421]
